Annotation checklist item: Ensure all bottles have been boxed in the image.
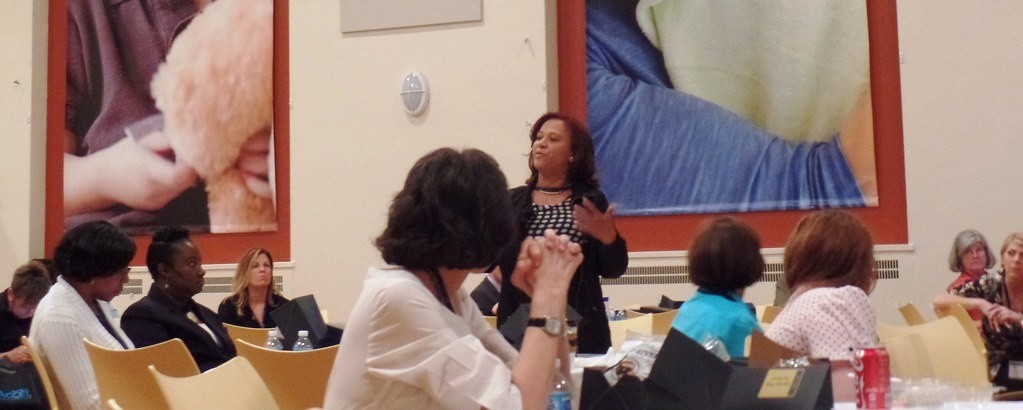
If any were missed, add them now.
[293,330,313,352]
[265,330,284,350]
[548,358,572,410]
[603,297,612,321]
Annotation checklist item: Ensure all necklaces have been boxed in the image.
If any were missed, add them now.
[538,183,569,195]
[533,183,572,191]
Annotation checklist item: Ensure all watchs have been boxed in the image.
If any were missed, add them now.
[526,314,565,335]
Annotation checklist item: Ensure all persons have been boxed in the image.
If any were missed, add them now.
[644,211,1023,410]
[471,262,502,316]
[64,0,275,233]
[496,113,629,354]
[323,147,584,410]
[0,220,292,410]
[586,0,879,213]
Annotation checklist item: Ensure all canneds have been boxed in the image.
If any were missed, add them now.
[852,344,893,409]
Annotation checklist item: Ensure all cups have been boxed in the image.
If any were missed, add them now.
[124,115,175,163]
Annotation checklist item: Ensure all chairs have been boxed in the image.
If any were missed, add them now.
[22,298,987,410]
